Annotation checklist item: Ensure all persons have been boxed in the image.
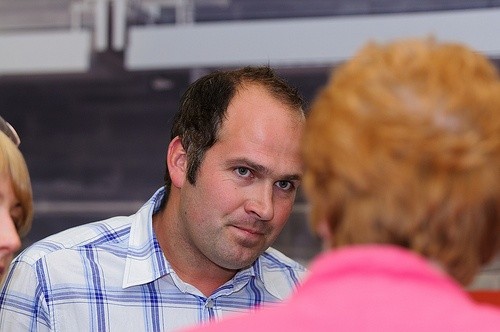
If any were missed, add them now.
[0,116,34,292]
[0,56,314,332]
[175,33,500,332]
